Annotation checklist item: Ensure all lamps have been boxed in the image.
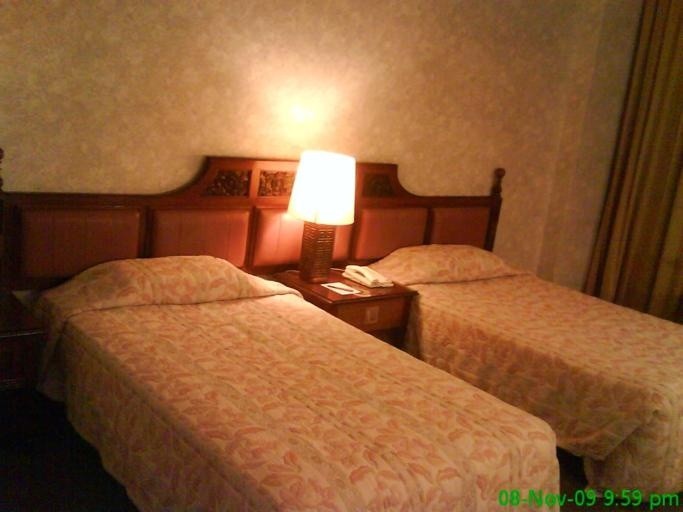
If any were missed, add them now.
[284,147,357,283]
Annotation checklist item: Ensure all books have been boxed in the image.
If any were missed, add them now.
[320,281,361,297]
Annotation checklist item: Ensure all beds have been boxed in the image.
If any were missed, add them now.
[31,251,566,512]
[364,243,681,499]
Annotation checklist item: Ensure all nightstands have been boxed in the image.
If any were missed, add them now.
[272,266,419,340]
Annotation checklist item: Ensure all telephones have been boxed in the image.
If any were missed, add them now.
[345,265,394,287]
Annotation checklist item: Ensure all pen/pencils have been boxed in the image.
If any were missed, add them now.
[327,286,353,292]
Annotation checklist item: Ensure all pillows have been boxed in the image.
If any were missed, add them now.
[386,244,517,286]
[87,255,253,310]
